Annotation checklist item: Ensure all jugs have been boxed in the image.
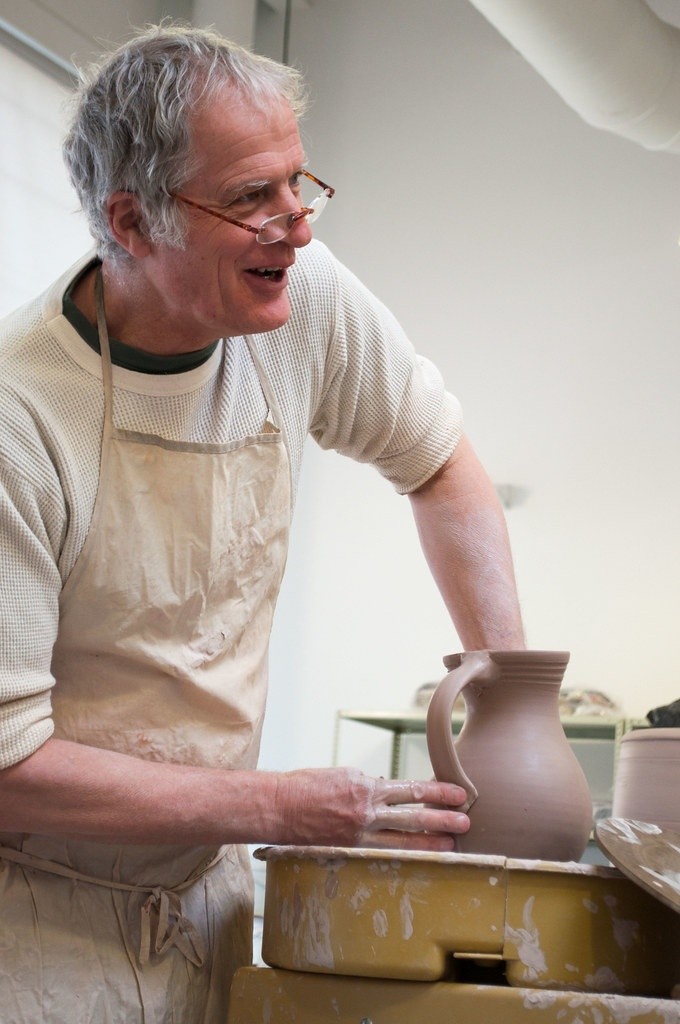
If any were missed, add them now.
[423,650,597,864]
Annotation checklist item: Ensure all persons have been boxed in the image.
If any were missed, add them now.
[0,28,525,1024]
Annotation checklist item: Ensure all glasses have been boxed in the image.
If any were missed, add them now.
[160,168,336,246]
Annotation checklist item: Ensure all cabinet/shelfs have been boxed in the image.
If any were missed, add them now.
[331,709,651,868]
